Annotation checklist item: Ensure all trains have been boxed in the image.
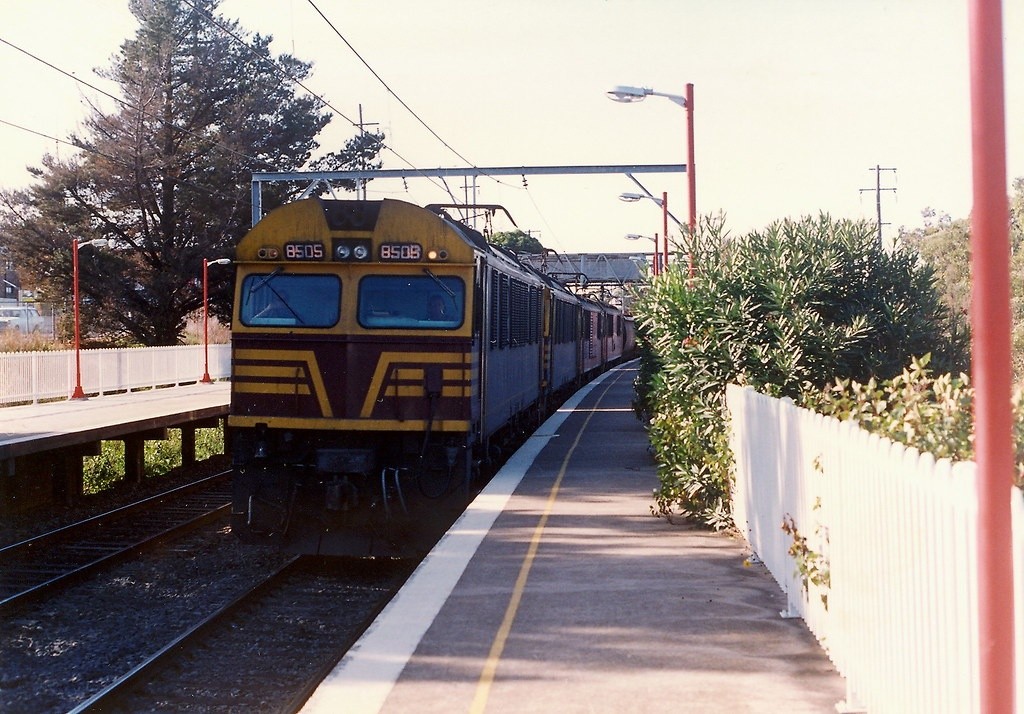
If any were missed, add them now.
[226,196,638,532]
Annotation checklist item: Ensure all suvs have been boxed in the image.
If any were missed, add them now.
[0,307,44,334]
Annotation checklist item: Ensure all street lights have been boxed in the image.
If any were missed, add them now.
[70,238,107,400]
[604,82,701,290]
[619,191,669,274]
[198,259,231,384]
[623,232,659,277]
